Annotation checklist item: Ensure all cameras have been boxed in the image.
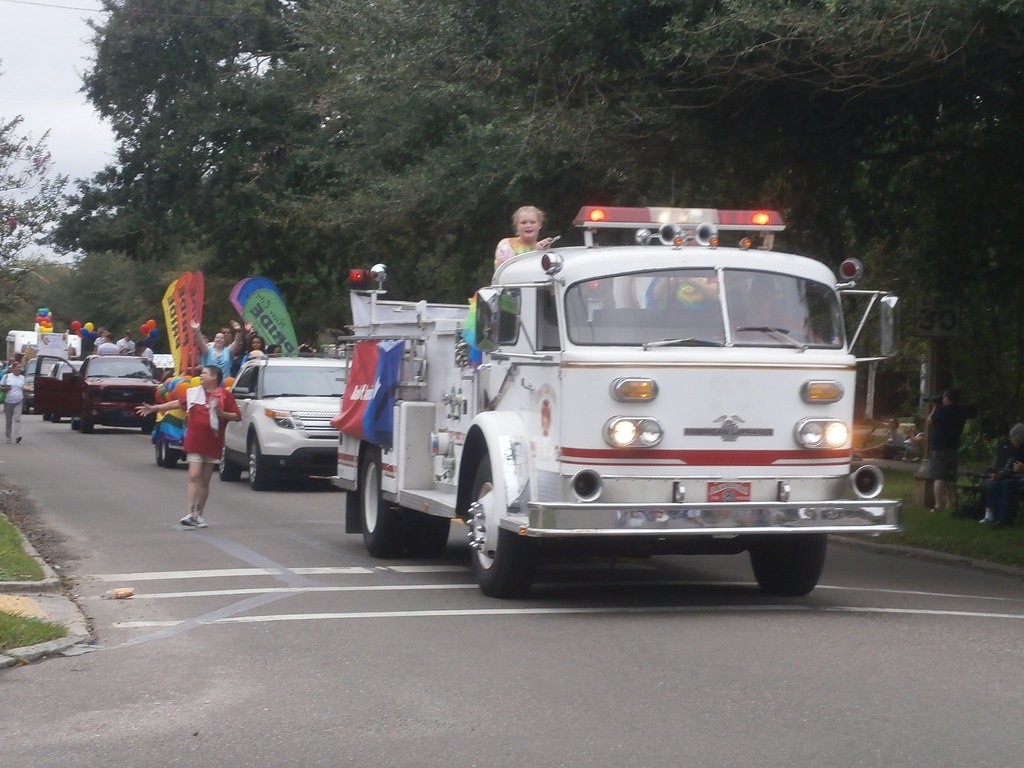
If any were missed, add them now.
[924,396,943,403]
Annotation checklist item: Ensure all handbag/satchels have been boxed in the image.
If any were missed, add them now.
[0,390,7,404]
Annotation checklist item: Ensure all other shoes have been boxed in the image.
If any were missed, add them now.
[929,508,942,513]
[901,457,907,462]
[855,449,869,458]
[912,457,921,463]
[979,518,992,524]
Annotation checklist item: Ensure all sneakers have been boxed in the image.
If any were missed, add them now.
[6,438,11,444]
[16,437,22,444]
[180,513,208,528]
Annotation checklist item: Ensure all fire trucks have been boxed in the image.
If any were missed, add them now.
[310,206,914,599]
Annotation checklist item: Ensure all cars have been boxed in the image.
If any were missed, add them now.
[33,354,165,434]
[154,375,235,468]
[218,350,353,492]
[152,353,175,383]
[21,358,61,414]
[43,360,85,423]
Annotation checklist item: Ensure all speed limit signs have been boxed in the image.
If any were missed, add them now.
[909,292,964,338]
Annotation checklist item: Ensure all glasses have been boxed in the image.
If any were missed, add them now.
[222,332,231,335]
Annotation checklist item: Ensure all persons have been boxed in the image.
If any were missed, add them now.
[854,389,1024,528]
[92,327,154,358]
[189,319,281,382]
[134,365,241,528]
[494,206,552,271]
[730,277,823,344]
[0,358,25,444]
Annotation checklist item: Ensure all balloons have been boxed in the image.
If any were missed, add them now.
[140,319,158,345]
[155,375,201,403]
[35,307,53,332]
[223,377,235,386]
[71,320,96,341]
